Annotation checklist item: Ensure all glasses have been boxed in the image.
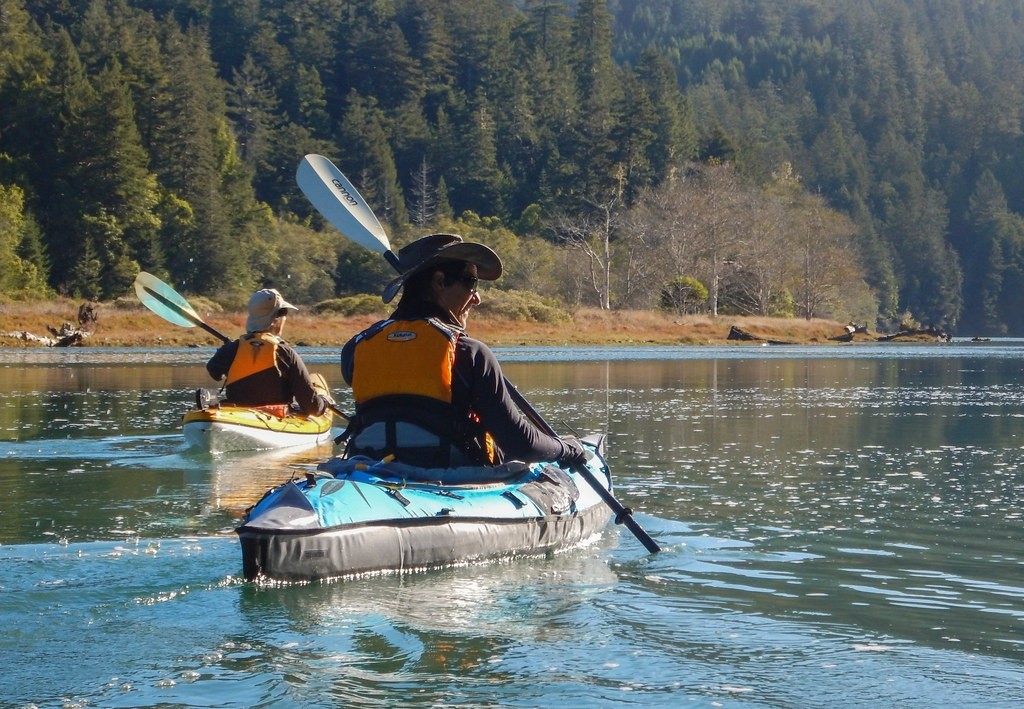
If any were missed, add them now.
[445,273,478,293]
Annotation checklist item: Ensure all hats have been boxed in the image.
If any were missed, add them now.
[247,289,299,333]
[382,233,503,304]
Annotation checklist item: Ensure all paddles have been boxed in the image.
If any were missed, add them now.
[133,270,353,425]
[293,151,663,556]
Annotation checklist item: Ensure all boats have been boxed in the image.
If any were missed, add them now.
[181,373,335,456]
[235,430,613,587]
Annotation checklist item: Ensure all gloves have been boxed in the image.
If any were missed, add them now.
[553,435,587,474]
[318,394,337,408]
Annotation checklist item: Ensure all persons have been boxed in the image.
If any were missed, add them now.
[206,289,338,417]
[340,233,588,474]
